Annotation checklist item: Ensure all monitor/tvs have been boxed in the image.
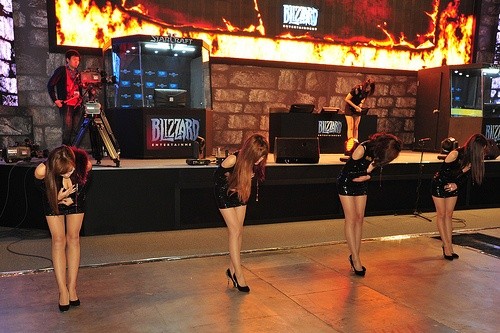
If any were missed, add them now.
[289,104,315,113]
[318,107,340,114]
[360,107,371,115]
[153,89,188,106]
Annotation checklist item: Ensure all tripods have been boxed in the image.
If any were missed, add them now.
[71,100,121,167]
[394,142,432,221]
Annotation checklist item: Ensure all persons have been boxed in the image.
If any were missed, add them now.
[431,133,487,260]
[336,132,402,276]
[35,145,92,311]
[48,49,83,146]
[344,77,375,140]
[213,133,269,292]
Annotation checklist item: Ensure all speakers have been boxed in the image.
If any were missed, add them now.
[483,139,500,160]
[274,137,319,164]
[2,146,31,163]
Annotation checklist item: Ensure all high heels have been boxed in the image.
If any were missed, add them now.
[232,273,250,292]
[226,268,238,289]
[348,255,366,277]
[58,291,70,313]
[69,297,80,306]
[452,252,459,259]
[441,246,453,261]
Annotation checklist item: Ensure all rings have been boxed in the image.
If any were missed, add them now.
[450,188,452,190]
[449,184,451,187]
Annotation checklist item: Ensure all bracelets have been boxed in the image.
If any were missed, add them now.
[360,101,363,103]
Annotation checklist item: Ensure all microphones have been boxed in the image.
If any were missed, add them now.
[418,138,432,141]
